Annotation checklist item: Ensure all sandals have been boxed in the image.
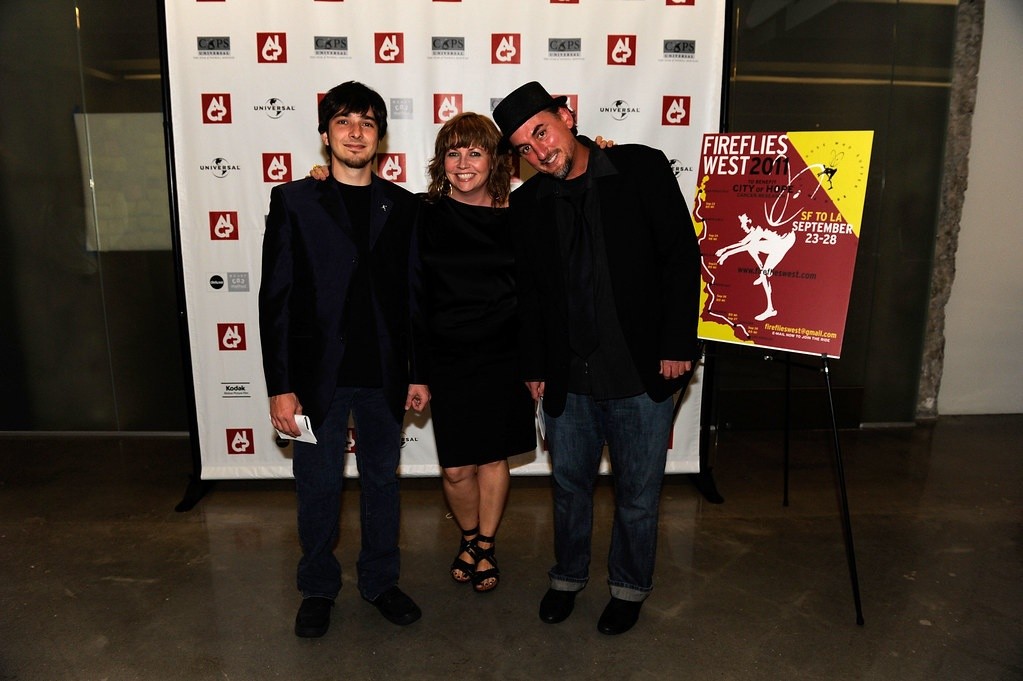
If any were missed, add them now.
[452,524,480,583]
[472,535,500,593]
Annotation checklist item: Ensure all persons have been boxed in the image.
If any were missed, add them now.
[258,81,432,637]
[493,87,701,634]
[311,111,612,592]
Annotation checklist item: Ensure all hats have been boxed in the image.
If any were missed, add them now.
[492,81,567,156]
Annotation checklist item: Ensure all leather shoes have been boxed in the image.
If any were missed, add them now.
[539,586,578,623]
[360,585,421,626]
[295,596,332,638]
[598,596,646,635]
